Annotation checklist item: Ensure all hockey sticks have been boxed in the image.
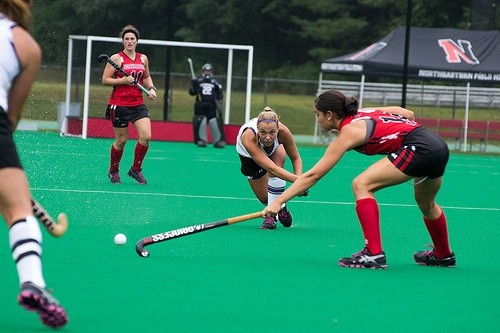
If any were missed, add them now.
[98,54,150,96]
[187,57,202,102]
[136,211,265,258]
[29,197,67,235]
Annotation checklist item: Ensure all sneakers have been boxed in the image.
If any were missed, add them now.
[339,245,387,270]
[108,169,120,182]
[260,217,277,229]
[414,248,456,267]
[16,282,66,327]
[127,167,146,183]
[278,207,292,227]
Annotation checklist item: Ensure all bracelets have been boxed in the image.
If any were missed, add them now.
[149,86,156,91]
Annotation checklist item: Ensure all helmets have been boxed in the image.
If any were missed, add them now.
[202,63,213,78]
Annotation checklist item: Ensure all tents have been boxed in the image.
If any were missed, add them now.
[313,26,500,153]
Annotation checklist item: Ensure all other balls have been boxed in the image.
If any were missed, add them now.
[114,233,127,245]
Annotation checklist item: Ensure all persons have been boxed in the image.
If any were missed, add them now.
[101,25,157,184]
[189,63,226,149]
[0,0,68,328]
[262,88,456,270]
[236,106,309,230]
